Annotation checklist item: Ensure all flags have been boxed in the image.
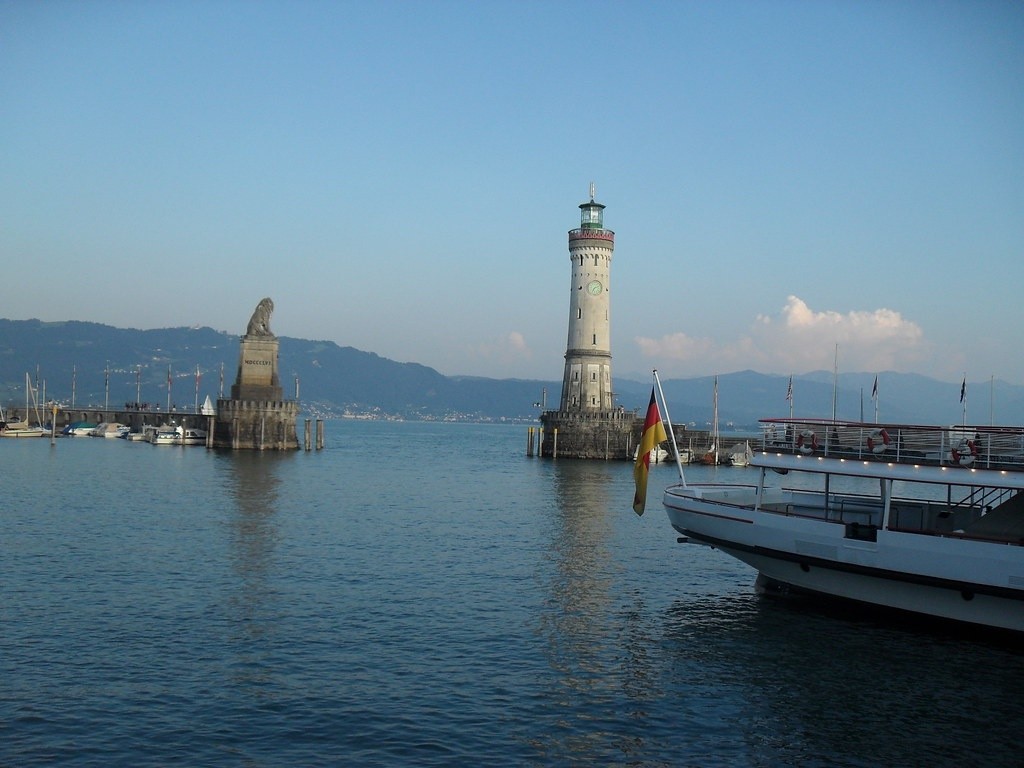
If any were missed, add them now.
[786,378,793,400]
[872,375,877,397]
[960,379,965,403]
[36,365,224,389]
[633,387,667,516]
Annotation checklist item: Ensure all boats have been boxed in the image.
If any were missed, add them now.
[662,418,1024,648]
[62,421,207,445]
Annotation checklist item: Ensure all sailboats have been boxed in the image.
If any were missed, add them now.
[0,371,44,437]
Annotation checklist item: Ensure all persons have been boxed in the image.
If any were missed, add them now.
[974,434,983,453]
[770,425,777,440]
[785,426,792,441]
[172,404,176,412]
[831,428,839,445]
[157,404,160,412]
[199,404,203,413]
[125,401,147,411]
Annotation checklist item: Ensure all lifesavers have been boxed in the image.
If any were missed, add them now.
[952,438,976,464]
[867,428,890,452]
[798,430,818,453]
